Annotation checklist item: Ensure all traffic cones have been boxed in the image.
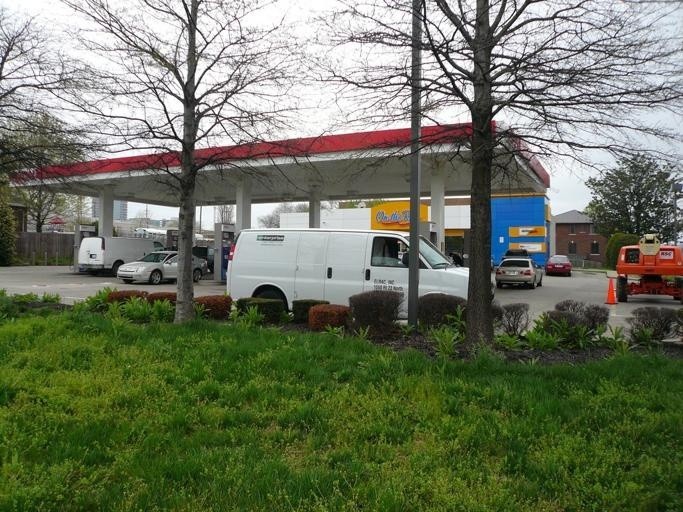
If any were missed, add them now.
[604,276,618,305]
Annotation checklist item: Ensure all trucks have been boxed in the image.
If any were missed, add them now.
[223,227,494,326]
[76,234,168,276]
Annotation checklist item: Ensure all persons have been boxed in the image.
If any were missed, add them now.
[448,252,463,267]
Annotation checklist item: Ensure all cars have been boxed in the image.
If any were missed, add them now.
[114,251,209,286]
[494,246,574,290]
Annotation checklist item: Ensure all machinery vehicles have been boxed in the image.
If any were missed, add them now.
[616,233,683,303]
